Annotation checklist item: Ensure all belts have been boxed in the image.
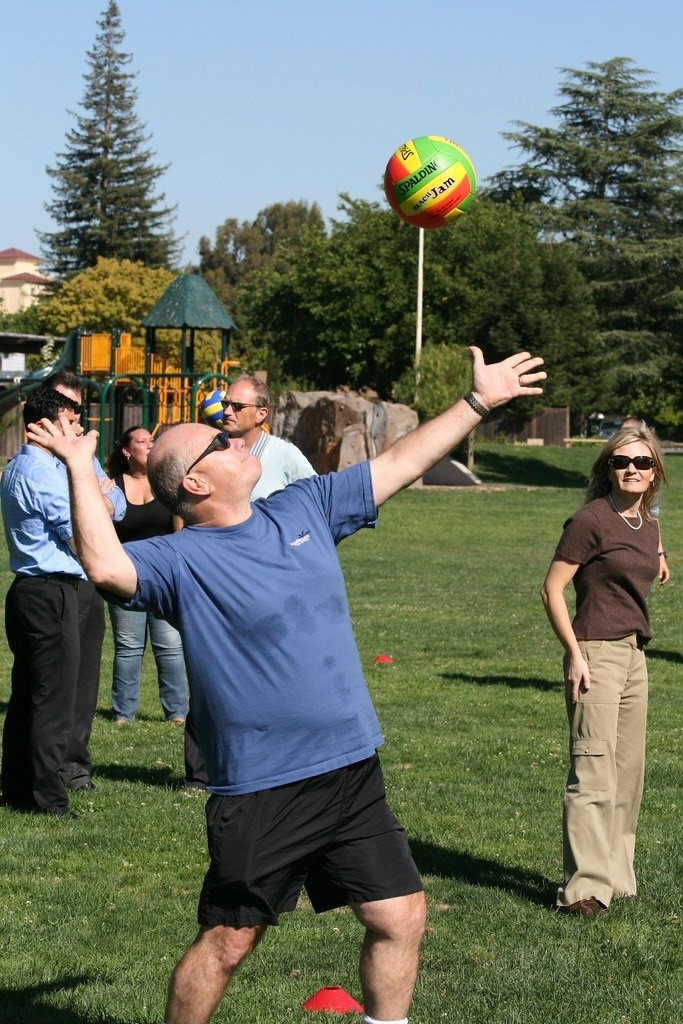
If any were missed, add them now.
[34,571,82,587]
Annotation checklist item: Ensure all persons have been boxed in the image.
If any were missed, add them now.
[220,375,318,505]
[0,365,192,818]
[27,341,548,1024]
[542,420,670,918]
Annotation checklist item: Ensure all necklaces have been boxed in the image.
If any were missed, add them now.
[608,489,643,530]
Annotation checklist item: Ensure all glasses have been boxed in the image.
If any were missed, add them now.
[186,432,230,474]
[221,400,264,411]
[608,455,655,470]
[74,405,85,414]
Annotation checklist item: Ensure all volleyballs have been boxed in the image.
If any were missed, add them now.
[383,135,478,232]
[199,391,233,427]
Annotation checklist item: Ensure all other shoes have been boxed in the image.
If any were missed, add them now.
[76,782,97,792]
[115,719,125,726]
[173,718,185,725]
[53,808,78,819]
[568,897,608,918]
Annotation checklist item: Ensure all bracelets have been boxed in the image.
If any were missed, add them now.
[658,552,668,559]
[465,391,489,418]
